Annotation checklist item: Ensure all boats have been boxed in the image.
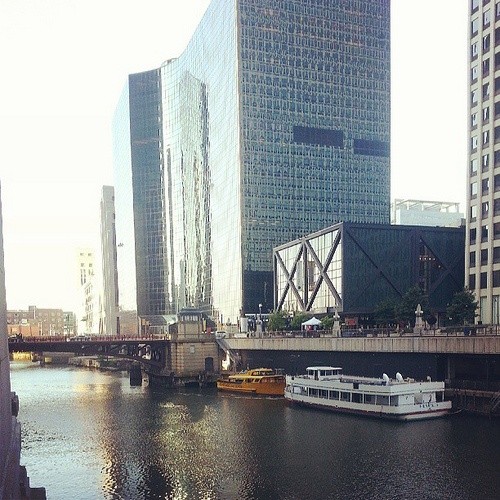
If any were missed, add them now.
[215,367,287,398]
[283,365,452,423]
